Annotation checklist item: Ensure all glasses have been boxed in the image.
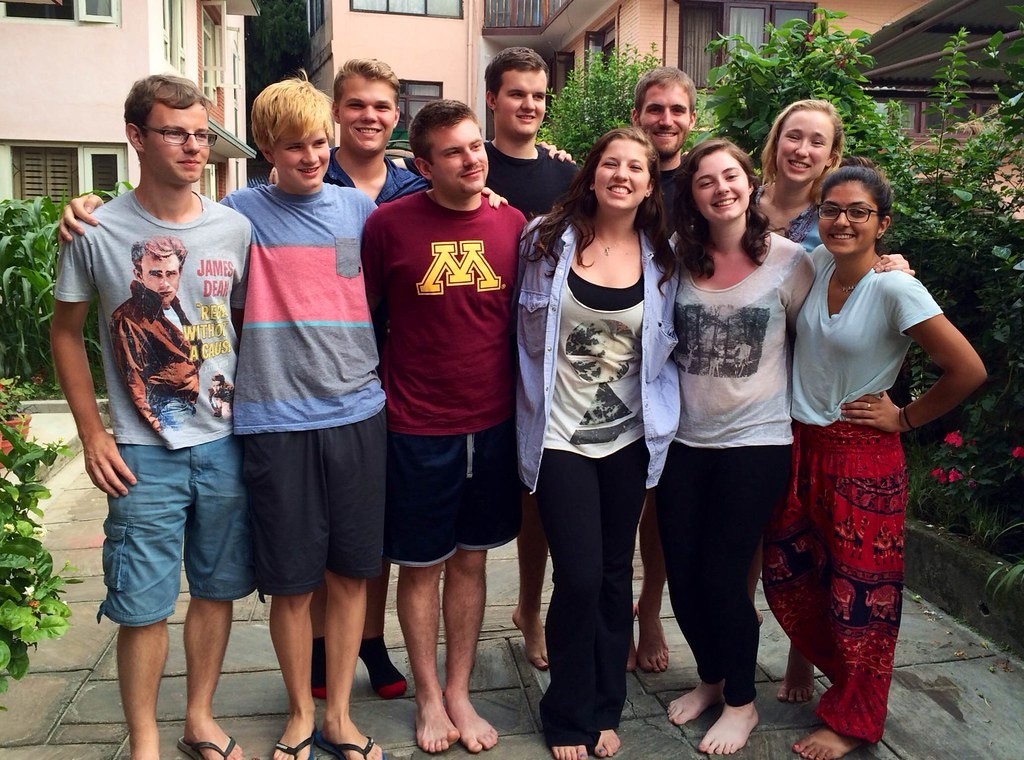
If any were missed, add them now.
[817,203,878,224]
[144,125,219,146]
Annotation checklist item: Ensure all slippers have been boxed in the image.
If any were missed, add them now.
[275,727,317,760]
[315,730,386,760]
[177,735,237,760]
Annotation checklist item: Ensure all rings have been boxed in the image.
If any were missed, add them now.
[868,403,871,407]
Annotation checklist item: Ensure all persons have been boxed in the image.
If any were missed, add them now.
[59,47,988,760]
[50,75,251,760]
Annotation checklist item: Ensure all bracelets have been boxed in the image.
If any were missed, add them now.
[904,407,917,430]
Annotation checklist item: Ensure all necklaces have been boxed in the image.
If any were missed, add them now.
[595,234,633,256]
[841,283,856,293]
